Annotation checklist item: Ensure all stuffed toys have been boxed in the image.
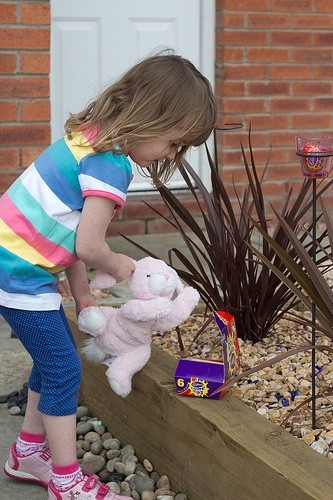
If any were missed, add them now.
[78,255,200,399]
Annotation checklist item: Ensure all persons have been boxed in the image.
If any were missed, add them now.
[0,47,219,500]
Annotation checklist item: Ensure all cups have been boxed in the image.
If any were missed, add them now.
[296,135,333,179]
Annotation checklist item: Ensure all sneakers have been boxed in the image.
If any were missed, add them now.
[48,471,134,500]
[2,442,53,490]
